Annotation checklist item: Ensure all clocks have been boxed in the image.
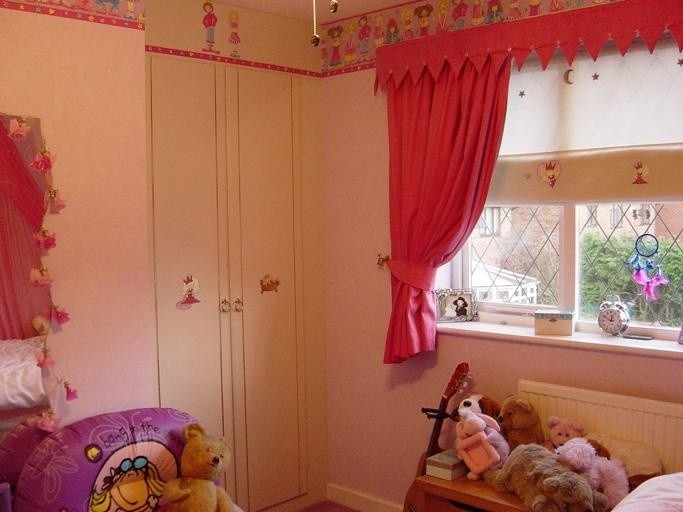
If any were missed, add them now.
[596,295,631,336]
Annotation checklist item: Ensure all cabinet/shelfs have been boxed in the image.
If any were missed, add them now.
[147,50,325,512]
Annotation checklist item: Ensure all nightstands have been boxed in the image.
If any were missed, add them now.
[402,475,525,512]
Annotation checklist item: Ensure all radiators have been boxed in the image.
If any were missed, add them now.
[517,379,683,475]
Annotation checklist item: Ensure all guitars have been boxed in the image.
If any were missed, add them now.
[403,363,473,511]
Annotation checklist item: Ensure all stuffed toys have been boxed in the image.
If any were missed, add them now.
[152,423,244,511]
[452,391,628,512]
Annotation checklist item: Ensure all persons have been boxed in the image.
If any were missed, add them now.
[450,296,469,316]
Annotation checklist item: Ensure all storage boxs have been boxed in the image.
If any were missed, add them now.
[534,306,574,335]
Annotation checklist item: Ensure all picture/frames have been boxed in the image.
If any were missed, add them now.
[432,286,480,323]
